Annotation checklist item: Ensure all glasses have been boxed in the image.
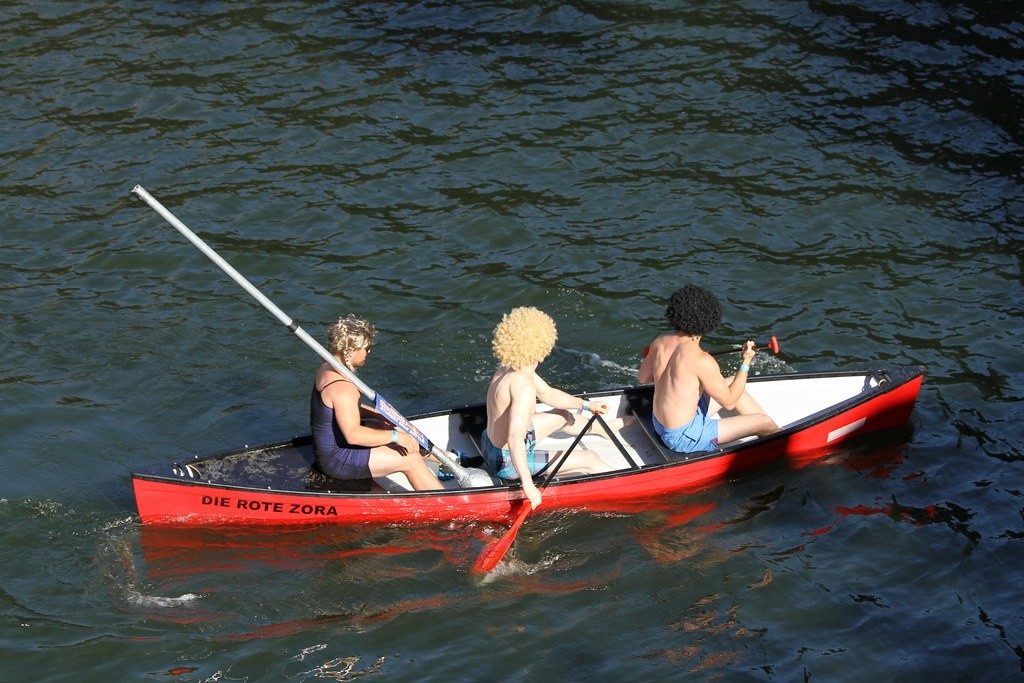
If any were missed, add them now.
[355,345,370,352]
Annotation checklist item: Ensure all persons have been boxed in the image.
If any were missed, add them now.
[638,284,777,453]
[482,306,635,510]
[311,316,484,492]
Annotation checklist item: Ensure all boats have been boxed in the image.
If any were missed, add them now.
[129,362,925,526]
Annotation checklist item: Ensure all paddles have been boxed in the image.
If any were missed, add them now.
[643,336,781,358]
[470,406,608,574]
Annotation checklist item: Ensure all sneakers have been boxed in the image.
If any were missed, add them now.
[439,448,486,481]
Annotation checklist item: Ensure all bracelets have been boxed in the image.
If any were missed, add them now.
[583,401,589,410]
[392,430,398,445]
[738,365,750,371]
